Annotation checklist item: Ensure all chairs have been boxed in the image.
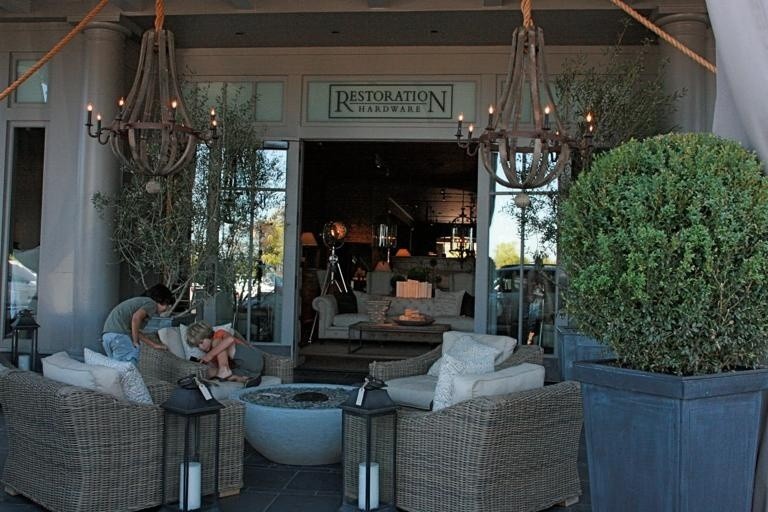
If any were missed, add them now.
[0,322,292,511]
[338,330,582,511]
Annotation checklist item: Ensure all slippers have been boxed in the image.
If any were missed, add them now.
[209,374,232,383]
[244,375,262,389]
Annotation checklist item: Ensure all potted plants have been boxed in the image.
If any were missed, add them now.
[561,132,767,512]
[91,66,281,334]
[551,17,698,384]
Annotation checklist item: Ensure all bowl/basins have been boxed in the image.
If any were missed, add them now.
[390,312,435,325]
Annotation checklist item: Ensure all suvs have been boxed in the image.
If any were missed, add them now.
[493,263,571,334]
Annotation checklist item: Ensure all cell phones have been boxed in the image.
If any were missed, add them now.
[189,355,208,365]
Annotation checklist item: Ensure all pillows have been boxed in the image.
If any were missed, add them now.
[353,290,466,320]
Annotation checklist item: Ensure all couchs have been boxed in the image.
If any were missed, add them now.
[311,289,474,344]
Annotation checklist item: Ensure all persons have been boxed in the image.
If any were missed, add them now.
[101,283,175,368]
[185,320,264,388]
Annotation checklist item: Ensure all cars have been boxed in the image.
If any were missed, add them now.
[5,256,38,339]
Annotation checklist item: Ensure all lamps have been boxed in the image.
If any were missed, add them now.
[455,0,596,210]
[395,248,411,257]
[301,232,318,246]
[84,0,220,196]
[446,153,475,268]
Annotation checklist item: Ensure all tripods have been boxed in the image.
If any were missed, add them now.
[307,252,349,345]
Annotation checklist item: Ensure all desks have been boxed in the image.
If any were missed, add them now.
[237,383,360,463]
